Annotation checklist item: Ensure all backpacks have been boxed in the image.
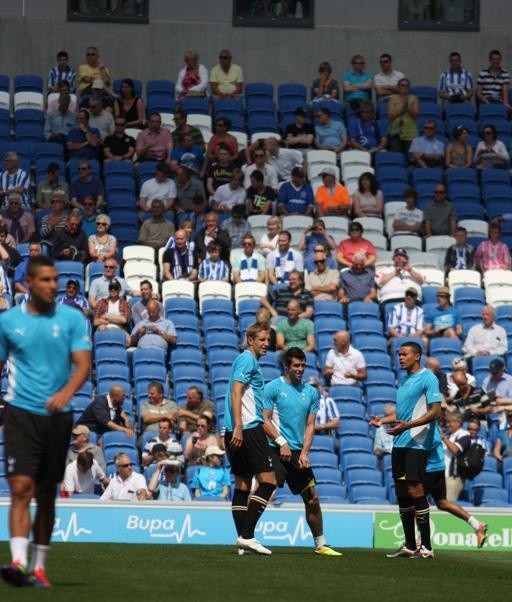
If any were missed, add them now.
[456,443,486,480]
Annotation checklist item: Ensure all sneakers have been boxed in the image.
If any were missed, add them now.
[407,544,435,560]
[312,544,343,555]
[0,561,53,588]
[236,535,273,555]
[474,521,488,548]
[384,546,419,558]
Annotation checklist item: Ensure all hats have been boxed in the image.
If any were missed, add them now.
[490,360,503,375]
[67,278,80,287]
[292,168,307,176]
[71,424,92,437]
[404,287,418,297]
[109,279,122,289]
[434,287,450,297]
[206,447,226,456]
[318,167,335,176]
[391,248,409,261]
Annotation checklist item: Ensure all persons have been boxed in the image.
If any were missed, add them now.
[59,377,231,503]
[1,254,95,589]
[0,46,512,358]
[370,341,442,560]
[245,346,343,556]
[222,321,278,554]
[414,421,488,550]
[304,331,511,501]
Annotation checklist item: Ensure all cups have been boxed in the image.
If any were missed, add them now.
[136,488,146,500]
[192,435,199,444]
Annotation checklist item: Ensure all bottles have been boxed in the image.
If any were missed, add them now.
[167,483,172,501]
